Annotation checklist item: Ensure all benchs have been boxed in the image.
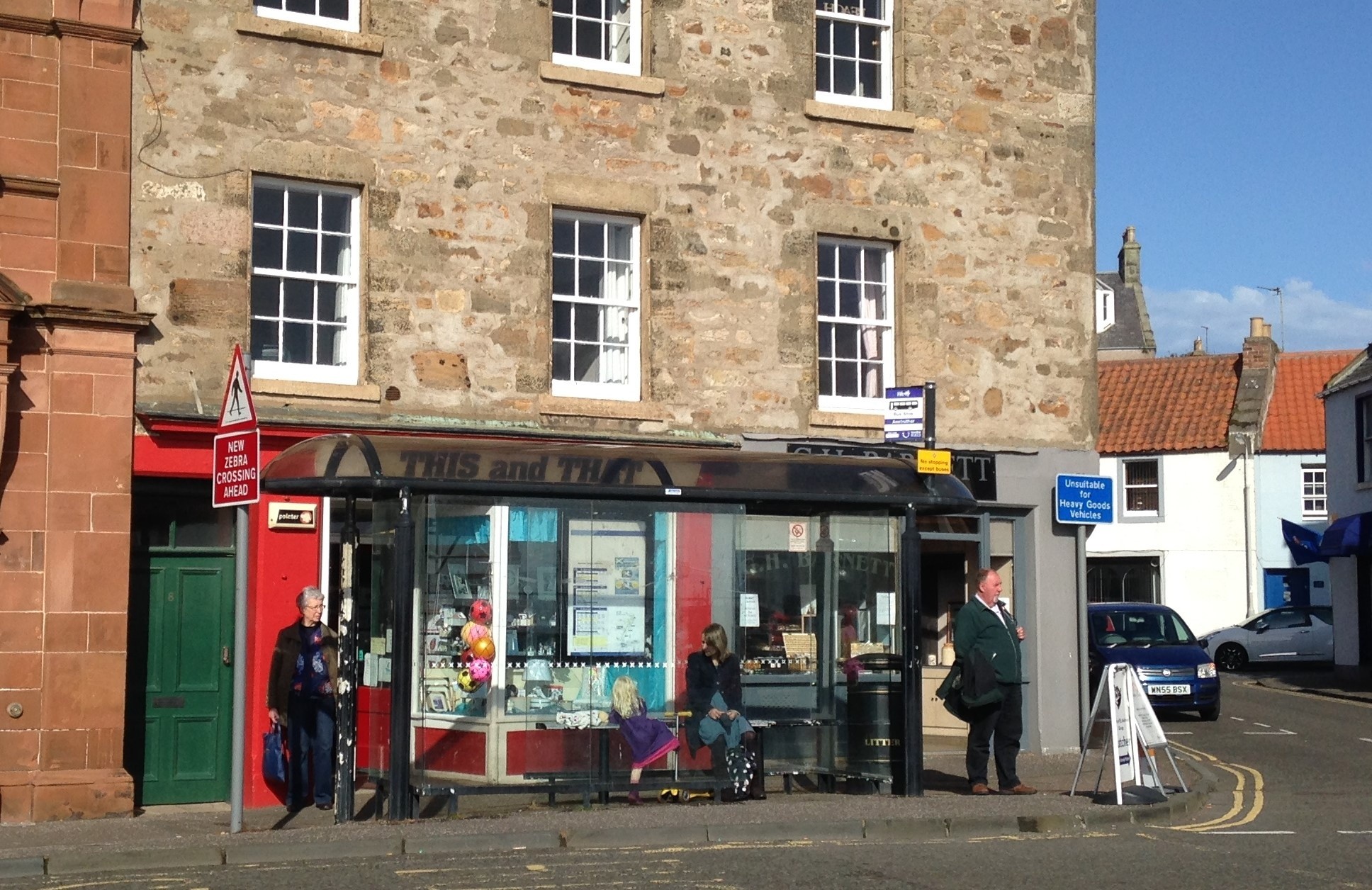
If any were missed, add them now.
[525,710,896,811]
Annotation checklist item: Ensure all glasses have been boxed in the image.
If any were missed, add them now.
[303,605,327,610]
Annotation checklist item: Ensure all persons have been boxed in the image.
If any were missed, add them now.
[267,586,338,812]
[953,569,1037,795]
[840,616,856,645]
[686,623,767,802]
[609,675,680,806]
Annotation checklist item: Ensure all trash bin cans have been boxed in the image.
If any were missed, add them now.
[841,652,909,793]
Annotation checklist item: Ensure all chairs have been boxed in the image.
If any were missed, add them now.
[1094,620,1127,645]
[782,630,823,676]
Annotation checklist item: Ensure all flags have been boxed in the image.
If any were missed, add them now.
[1281,519,1329,566]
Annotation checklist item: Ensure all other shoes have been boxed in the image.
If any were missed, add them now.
[287,799,299,812]
[628,791,644,805]
[316,802,332,810]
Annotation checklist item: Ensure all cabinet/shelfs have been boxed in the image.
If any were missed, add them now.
[373,494,892,794]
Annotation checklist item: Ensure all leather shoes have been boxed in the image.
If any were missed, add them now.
[972,783,989,795]
[720,788,749,801]
[749,786,767,800]
[999,782,1037,795]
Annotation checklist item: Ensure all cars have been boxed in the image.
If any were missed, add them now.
[1089,602,1222,722]
[1196,604,1334,674]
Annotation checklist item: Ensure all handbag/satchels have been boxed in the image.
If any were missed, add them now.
[263,717,286,784]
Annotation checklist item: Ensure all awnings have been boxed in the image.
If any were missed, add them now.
[1321,511,1372,557]
